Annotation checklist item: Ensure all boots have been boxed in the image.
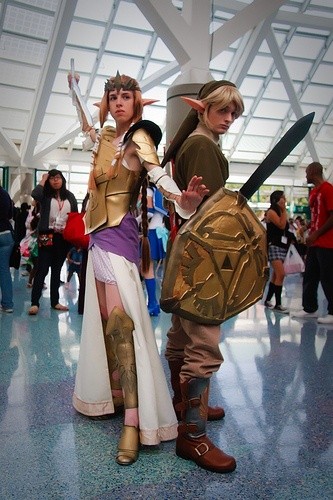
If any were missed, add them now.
[175,376,236,474]
[168,361,227,421]
[144,278,161,317]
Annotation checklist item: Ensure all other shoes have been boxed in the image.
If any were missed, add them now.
[273,304,289,313]
[291,309,319,318]
[2,306,14,312]
[116,425,139,466]
[264,301,274,310]
[54,302,69,311]
[317,315,333,323]
[29,306,39,314]
[27,282,32,287]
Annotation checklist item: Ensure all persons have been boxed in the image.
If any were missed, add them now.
[160,79,245,473]
[14,202,29,270]
[21,198,40,276]
[28,168,78,314]
[26,232,47,289]
[136,186,170,316]
[162,199,187,286]
[68,70,210,465]
[64,248,81,290]
[291,162,333,323]
[0,185,15,312]
[264,190,290,311]
[289,216,307,276]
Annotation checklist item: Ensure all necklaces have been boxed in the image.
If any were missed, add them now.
[57,200,64,211]
[194,130,220,147]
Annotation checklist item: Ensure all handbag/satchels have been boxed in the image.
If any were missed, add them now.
[19,232,37,256]
[63,212,91,249]
[282,244,306,273]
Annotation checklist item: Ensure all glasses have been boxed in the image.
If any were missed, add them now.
[49,175,62,179]
